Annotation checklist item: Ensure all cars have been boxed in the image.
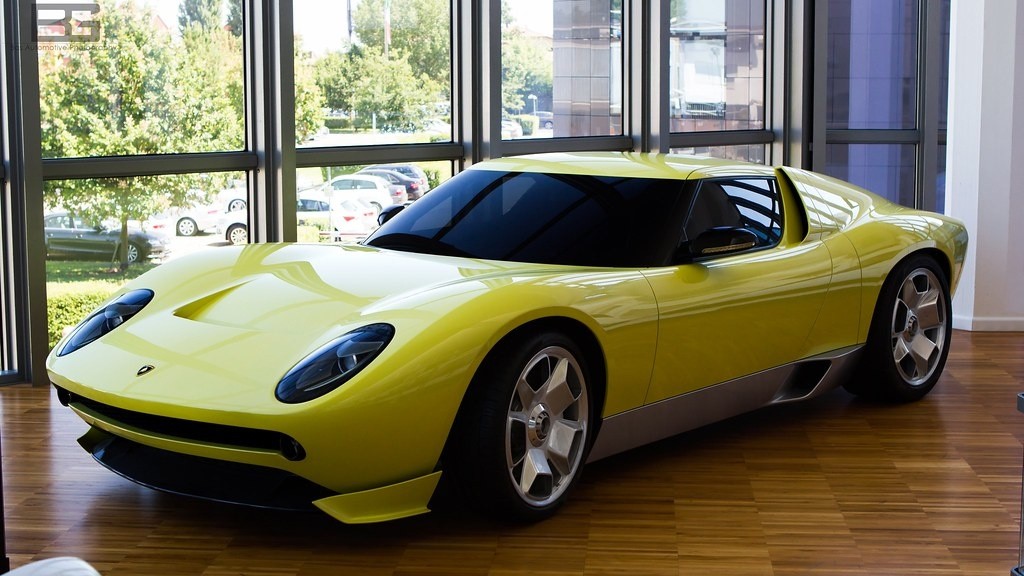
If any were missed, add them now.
[529,111,554,130]
[42,153,438,266]
[669,17,763,122]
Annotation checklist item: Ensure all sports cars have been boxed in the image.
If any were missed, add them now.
[41,146,975,532]
